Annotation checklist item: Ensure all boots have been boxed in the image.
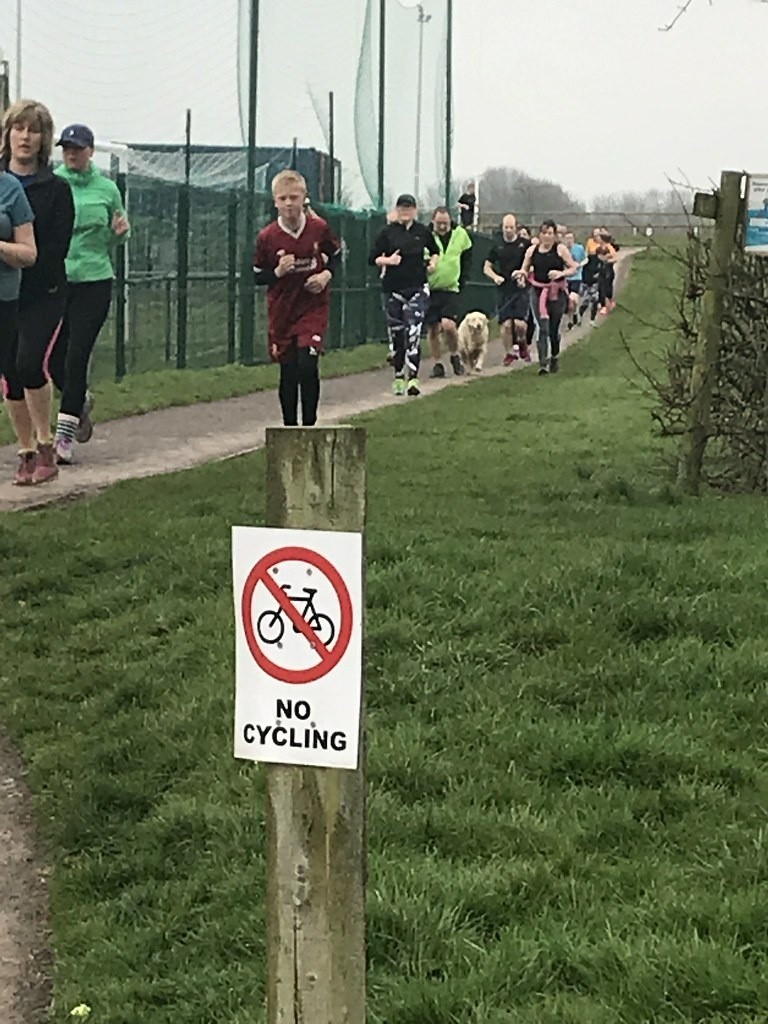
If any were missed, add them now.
[12,450,37,485]
[30,442,59,483]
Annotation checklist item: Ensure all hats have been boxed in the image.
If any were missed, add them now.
[396,194,416,207]
[55,124,94,147]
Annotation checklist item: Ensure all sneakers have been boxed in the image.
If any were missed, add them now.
[429,363,445,378]
[518,340,528,359]
[74,389,96,443]
[407,378,420,395]
[502,353,518,366]
[450,354,465,375]
[52,437,75,465]
[392,378,407,395]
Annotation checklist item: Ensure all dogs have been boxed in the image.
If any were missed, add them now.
[457,312,489,372]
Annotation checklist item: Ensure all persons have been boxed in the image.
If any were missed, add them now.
[483,214,620,374]
[424,207,473,377]
[254,171,341,426]
[457,184,478,230]
[0,99,76,486]
[369,194,440,396]
[54,124,130,466]
[0,121,37,374]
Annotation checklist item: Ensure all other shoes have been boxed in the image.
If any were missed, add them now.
[589,320,599,328]
[537,364,549,375]
[577,314,583,326]
[550,357,560,373]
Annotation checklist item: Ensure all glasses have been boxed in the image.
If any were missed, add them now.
[63,141,86,152]
